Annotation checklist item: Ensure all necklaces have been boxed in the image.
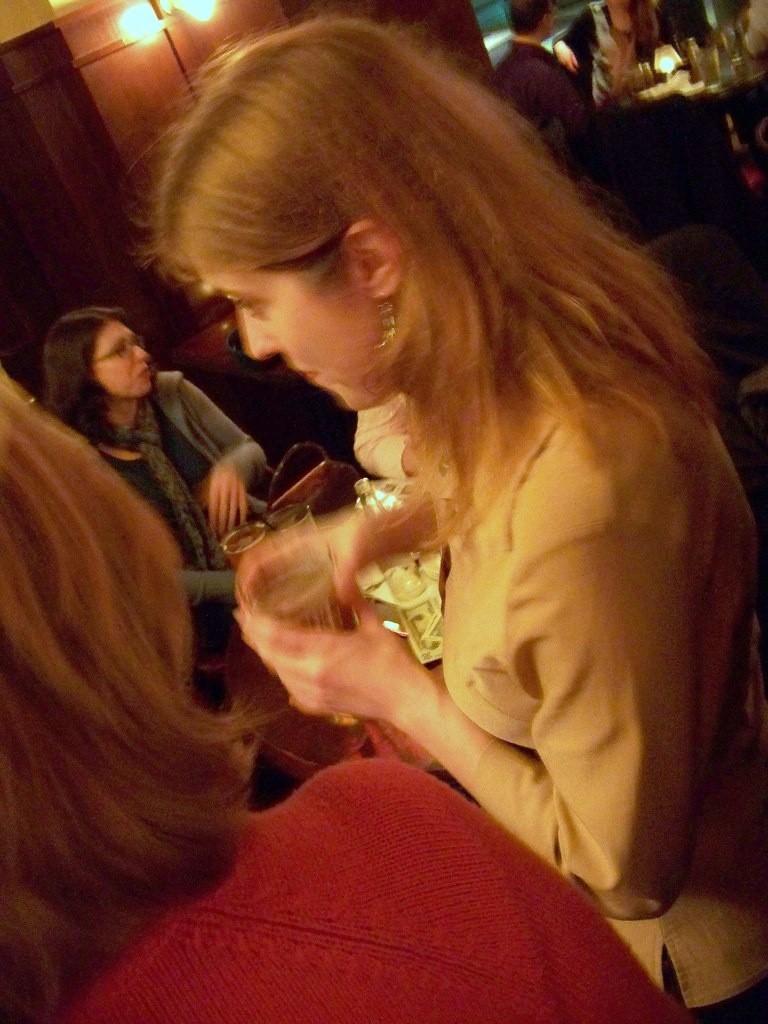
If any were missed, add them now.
[438,449,454,476]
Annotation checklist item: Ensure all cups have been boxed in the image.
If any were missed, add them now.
[219,522,269,570]
[269,504,317,533]
[237,538,361,726]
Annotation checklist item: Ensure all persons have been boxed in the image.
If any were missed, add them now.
[1,0,768,1024]
[148,15,768,1024]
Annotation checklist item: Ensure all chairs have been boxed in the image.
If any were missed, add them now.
[646,224,768,444]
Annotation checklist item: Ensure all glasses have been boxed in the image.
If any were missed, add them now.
[93,336,145,365]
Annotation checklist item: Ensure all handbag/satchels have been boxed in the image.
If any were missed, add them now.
[262,440,361,531]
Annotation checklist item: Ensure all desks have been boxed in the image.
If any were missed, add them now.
[586,71,768,278]
[222,623,472,796]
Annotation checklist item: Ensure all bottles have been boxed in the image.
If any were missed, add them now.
[354,477,426,603]
[685,36,702,69]
[633,61,654,89]
